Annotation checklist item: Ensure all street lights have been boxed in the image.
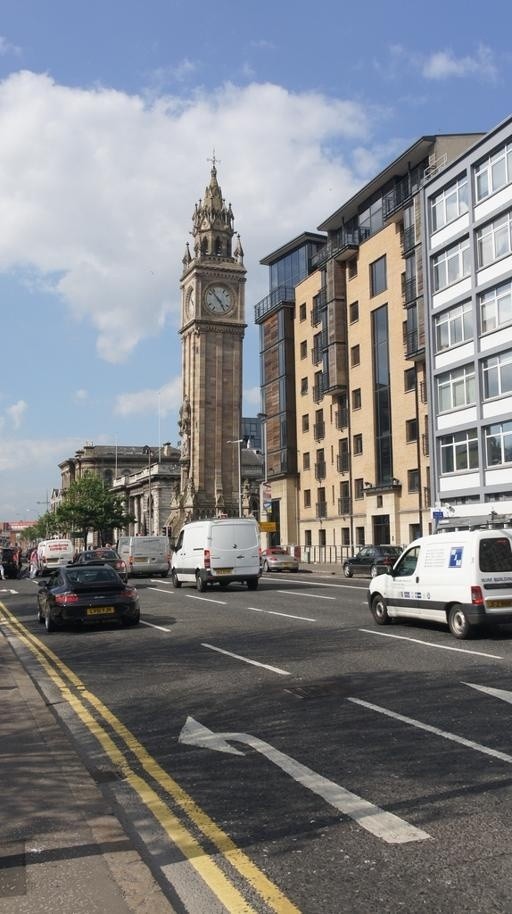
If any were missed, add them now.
[237,435,255,518]
[149,443,172,535]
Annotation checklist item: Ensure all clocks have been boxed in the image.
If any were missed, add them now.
[184,281,237,321]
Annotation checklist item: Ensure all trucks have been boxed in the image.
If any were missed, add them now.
[115,536,170,576]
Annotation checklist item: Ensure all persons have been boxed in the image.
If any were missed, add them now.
[210,166,217,177]
[0,548,32,579]
[73,542,117,559]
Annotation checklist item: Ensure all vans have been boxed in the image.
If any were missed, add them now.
[369,529,512,638]
[37,538,74,577]
[170,519,261,592]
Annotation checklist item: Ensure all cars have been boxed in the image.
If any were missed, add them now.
[343,544,404,579]
[68,549,129,585]
[260,549,299,571]
[36,564,140,631]
